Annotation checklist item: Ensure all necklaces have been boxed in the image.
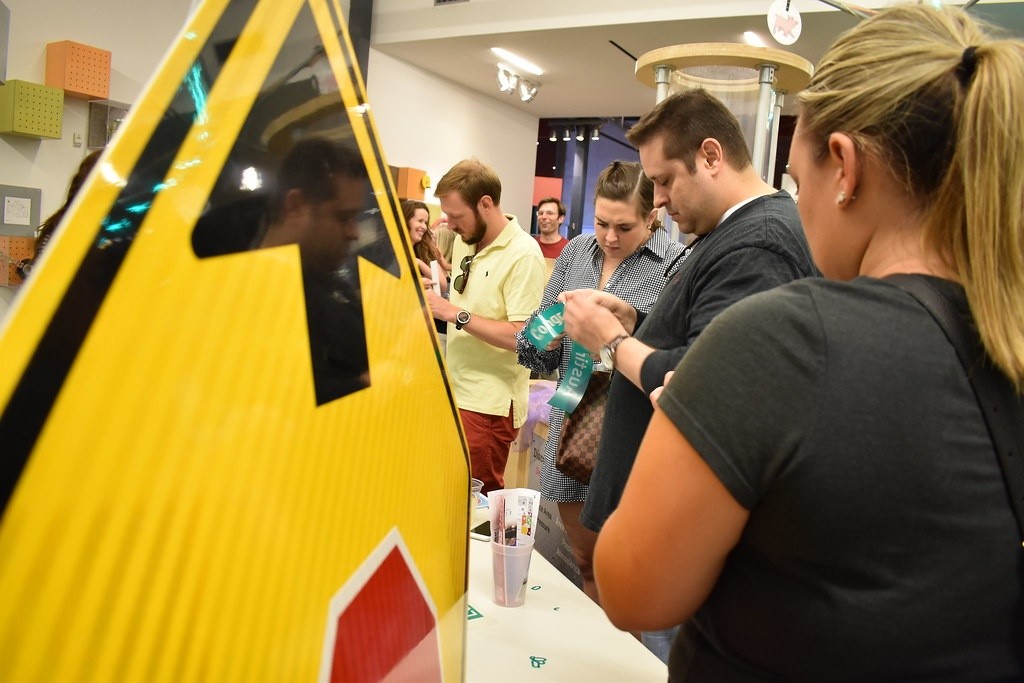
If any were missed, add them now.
[601,266,615,274]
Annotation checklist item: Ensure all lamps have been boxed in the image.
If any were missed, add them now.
[496,62,543,104]
[576,125,586,141]
[591,124,604,140]
[562,124,572,142]
[548,125,559,141]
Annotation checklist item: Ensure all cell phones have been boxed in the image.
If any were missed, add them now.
[470,520,491,542]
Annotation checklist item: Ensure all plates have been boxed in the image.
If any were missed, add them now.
[477,493,489,509]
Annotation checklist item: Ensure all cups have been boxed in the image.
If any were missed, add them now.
[491,537,534,607]
[472,478,484,529]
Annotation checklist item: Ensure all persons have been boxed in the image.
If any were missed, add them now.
[594,3,1023,683]
[401,200,459,299]
[557,87,826,663]
[420,158,544,495]
[533,197,570,259]
[253,140,367,261]
[35,149,104,256]
[516,159,693,604]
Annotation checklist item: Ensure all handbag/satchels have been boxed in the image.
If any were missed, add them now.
[555,370,613,486]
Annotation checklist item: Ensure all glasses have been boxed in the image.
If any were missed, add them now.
[664,232,709,278]
[454,254,475,294]
[536,211,559,217]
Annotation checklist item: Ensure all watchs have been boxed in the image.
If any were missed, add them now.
[456,310,470,330]
[599,334,628,370]
